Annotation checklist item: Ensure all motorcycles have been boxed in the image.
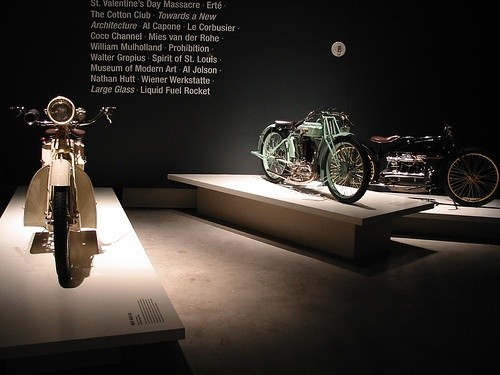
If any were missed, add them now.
[250,108,370,202]
[366,123,500,207]
[9,95,116,286]
